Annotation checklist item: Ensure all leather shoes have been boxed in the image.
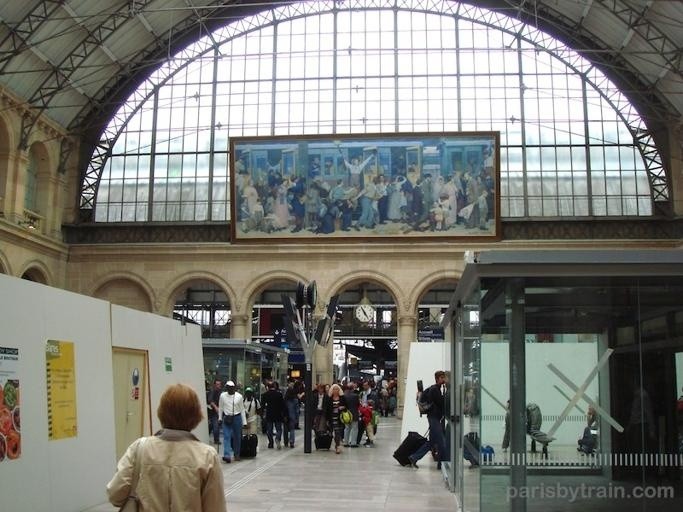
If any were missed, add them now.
[336,440,374,454]
[223,456,231,463]
[235,456,241,460]
[214,441,221,444]
[268,438,295,448]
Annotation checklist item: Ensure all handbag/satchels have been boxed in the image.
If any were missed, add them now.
[223,416,233,424]
[119,436,146,512]
[340,409,353,425]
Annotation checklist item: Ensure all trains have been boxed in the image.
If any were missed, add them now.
[233,138,492,204]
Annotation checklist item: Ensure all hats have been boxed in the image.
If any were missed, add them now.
[226,381,235,387]
[246,387,253,392]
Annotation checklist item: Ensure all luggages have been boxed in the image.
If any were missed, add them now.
[393,428,431,468]
[315,430,333,450]
[240,426,257,457]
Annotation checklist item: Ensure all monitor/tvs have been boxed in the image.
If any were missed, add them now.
[281,278,340,347]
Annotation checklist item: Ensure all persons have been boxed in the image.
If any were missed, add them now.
[443,371,452,419]
[500,399,531,453]
[575,402,600,452]
[104,382,228,511]
[234,142,493,233]
[207,373,398,463]
[620,376,656,469]
[407,370,447,470]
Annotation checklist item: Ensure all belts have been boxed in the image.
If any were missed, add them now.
[224,413,241,416]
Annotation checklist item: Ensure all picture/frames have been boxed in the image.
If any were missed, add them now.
[227,129,503,244]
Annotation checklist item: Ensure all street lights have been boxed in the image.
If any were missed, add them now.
[278,280,339,454]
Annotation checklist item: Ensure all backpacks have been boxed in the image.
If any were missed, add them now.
[417,385,441,417]
[371,409,380,425]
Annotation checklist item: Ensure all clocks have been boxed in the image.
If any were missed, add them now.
[355,302,374,324]
